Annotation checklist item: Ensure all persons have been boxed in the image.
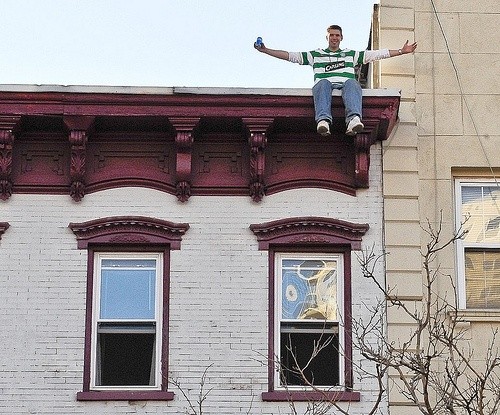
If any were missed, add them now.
[254,25,417,137]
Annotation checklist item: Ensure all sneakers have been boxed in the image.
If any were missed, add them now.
[316,120,330,136]
[346,116,365,134]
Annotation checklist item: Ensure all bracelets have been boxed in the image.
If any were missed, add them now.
[399,49,402,55]
